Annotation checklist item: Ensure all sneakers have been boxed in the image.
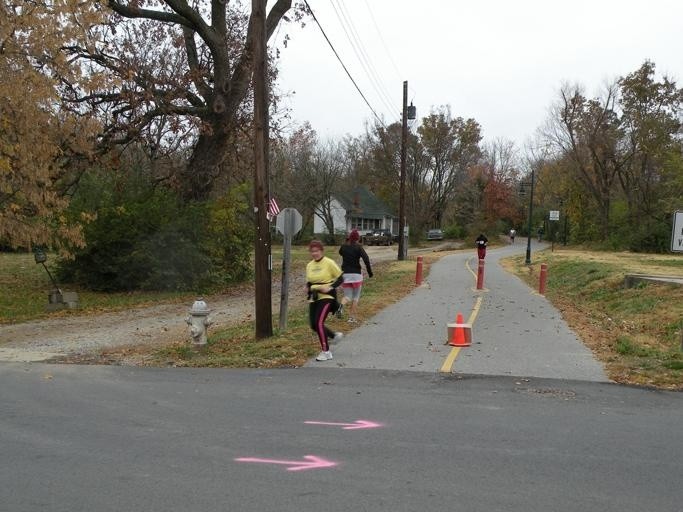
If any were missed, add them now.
[336,305,342,318]
[316,350,333,360]
[347,317,356,323]
[331,333,342,343]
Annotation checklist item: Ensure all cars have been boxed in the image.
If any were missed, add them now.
[425,229,445,241]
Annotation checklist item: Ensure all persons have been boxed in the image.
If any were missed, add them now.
[338,227,373,323]
[302,239,344,362]
[537,228,544,242]
[475,233,489,259]
[509,228,516,244]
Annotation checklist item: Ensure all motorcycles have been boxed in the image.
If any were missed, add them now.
[475,240,488,259]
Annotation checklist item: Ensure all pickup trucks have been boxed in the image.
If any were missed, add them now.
[366,229,395,246]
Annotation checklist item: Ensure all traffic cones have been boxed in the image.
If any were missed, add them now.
[449,314,468,347]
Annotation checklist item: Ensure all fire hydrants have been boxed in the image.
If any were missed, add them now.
[184,297,212,351]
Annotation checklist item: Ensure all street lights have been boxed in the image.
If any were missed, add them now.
[518,181,533,265]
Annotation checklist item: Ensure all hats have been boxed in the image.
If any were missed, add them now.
[349,230,359,241]
